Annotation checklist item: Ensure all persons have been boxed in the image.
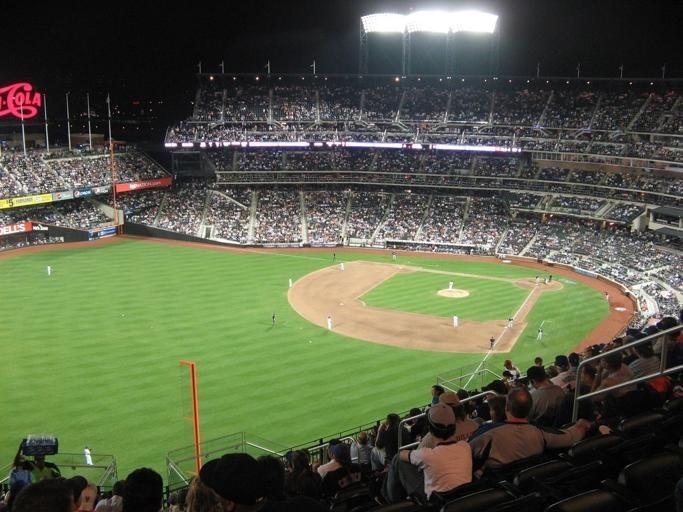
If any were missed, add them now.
[288,276,293,288]
[1,359,589,512]
[453,314,458,327]
[544,274,547,284]
[535,274,539,285]
[327,316,332,330]
[536,326,543,342]
[508,315,513,327]
[549,273,552,282]
[47,264,51,276]
[340,262,343,271]
[0,75,683,265]
[448,280,453,290]
[554,263,681,512]
[489,337,495,349]
[272,314,276,324]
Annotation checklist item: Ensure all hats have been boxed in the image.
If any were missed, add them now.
[438,392,460,407]
[429,402,456,427]
[332,443,348,458]
[504,359,512,366]
[123,467,164,501]
[285,450,307,469]
[554,355,569,368]
[198,451,268,506]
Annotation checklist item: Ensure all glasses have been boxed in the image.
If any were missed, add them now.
[425,408,448,432]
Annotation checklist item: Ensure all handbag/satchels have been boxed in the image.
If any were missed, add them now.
[618,381,661,417]
